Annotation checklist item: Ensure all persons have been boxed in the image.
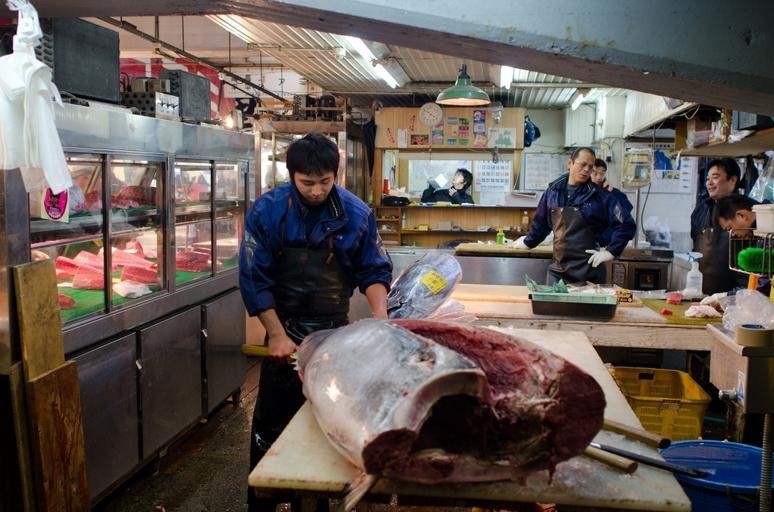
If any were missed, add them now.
[512,148,630,286]
[239,134,394,474]
[421,168,474,205]
[590,159,633,246]
[712,195,774,239]
[691,158,760,296]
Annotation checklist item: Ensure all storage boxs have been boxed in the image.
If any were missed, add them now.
[610,366,711,441]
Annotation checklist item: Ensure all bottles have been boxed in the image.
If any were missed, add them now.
[497,224,505,245]
[521,210,530,233]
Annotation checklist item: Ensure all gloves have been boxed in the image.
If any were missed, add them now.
[585,246,614,269]
[509,235,530,250]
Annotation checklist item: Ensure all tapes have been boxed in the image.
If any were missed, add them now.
[736,323,774,347]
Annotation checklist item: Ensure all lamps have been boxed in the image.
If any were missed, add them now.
[436,64,491,106]
[331,33,412,88]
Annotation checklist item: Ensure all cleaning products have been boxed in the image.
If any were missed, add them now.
[521,211,529,225]
[496,226,505,243]
[677,251,704,294]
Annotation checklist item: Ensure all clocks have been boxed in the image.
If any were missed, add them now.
[420,103,442,127]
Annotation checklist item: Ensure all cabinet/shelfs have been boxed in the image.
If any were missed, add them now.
[374,145,539,235]
[674,109,774,158]
